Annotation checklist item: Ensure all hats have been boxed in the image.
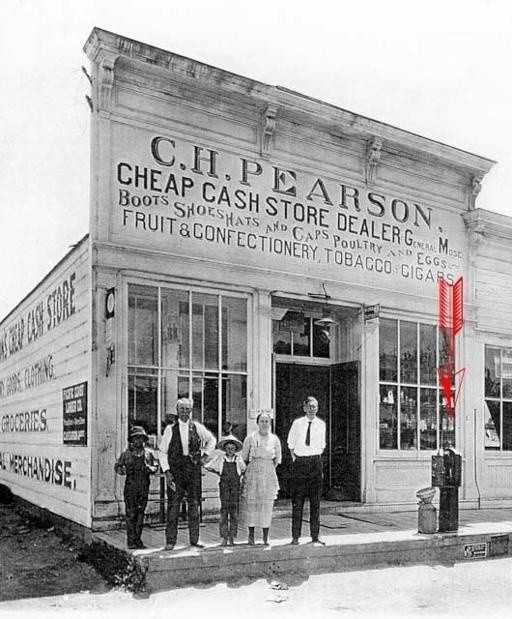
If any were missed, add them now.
[128,426,148,442]
[217,434,242,452]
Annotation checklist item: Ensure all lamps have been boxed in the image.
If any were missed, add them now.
[313,305,338,347]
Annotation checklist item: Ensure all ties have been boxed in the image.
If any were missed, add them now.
[305,421,312,446]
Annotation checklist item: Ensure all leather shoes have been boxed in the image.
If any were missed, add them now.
[129,539,326,550]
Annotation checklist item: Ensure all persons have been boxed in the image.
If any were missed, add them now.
[286,396,327,545]
[204,435,246,547]
[158,397,217,550]
[114,426,159,549]
[240,414,282,546]
